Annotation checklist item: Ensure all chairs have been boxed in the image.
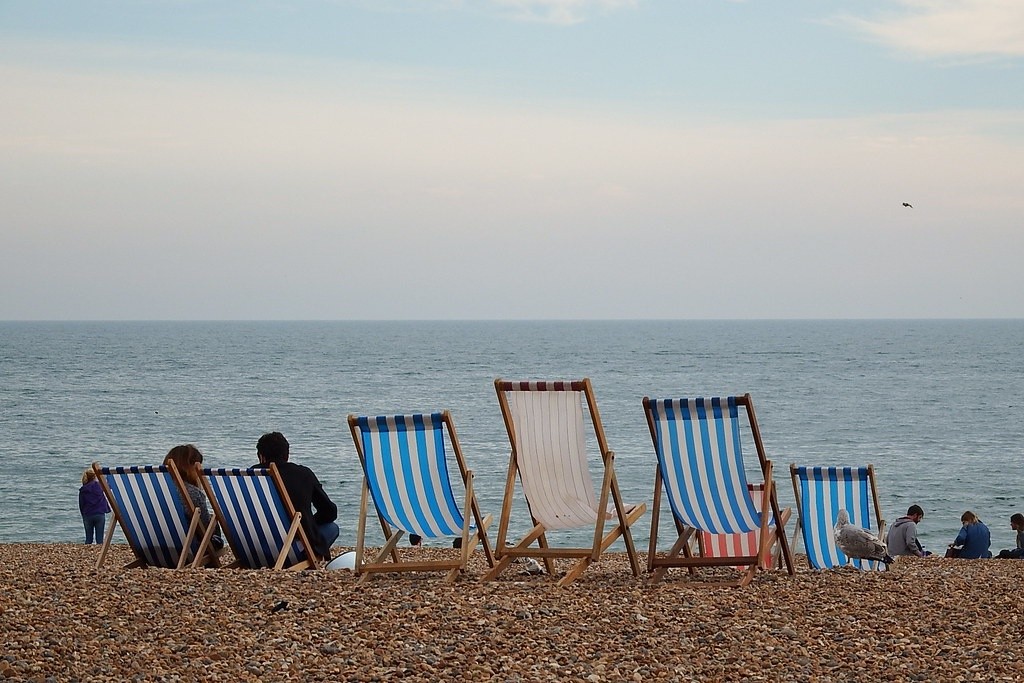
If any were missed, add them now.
[347,409,496,588]
[481,378,647,587]
[643,394,796,589]
[789,463,891,572]
[92,459,222,570]
[191,462,321,572]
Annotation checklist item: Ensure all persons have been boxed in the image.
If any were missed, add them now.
[452,537,462,548]
[162,444,224,569]
[948,511,992,559]
[79,468,112,544]
[250,431,339,562]
[408,533,422,548]
[885,505,932,557]
[1010,513,1024,559]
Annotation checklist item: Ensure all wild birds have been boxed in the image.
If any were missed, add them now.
[902,202,914,209]
[833,508,896,571]
